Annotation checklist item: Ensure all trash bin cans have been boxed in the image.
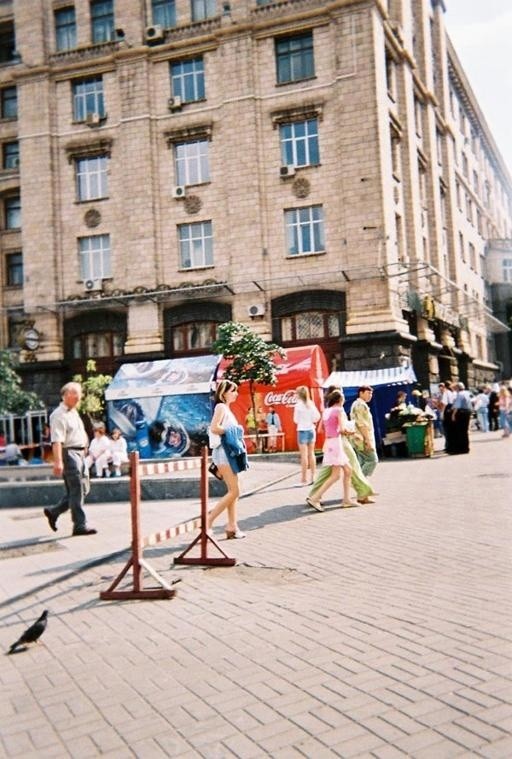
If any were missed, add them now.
[402,421,434,458]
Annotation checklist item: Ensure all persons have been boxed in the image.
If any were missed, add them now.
[305,383,380,505]
[206,379,248,539]
[307,392,362,511]
[350,385,378,478]
[292,386,321,487]
[0,423,130,478]
[387,379,512,454]
[244,405,283,453]
[43,381,98,535]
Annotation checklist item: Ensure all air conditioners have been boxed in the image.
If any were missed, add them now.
[141,22,165,45]
[165,93,183,110]
[277,162,295,180]
[170,185,186,197]
[246,301,265,320]
[10,155,20,169]
[83,110,100,128]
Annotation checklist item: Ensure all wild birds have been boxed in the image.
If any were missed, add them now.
[9,609,49,654]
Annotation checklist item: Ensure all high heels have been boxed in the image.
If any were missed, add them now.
[225,528,244,539]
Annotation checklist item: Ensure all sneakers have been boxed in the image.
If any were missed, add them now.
[307,499,323,511]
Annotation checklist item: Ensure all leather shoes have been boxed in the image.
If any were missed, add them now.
[44,507,58,531]
[73,529,96,535]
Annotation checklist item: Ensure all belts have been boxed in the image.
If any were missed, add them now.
[68,446,85,451]
